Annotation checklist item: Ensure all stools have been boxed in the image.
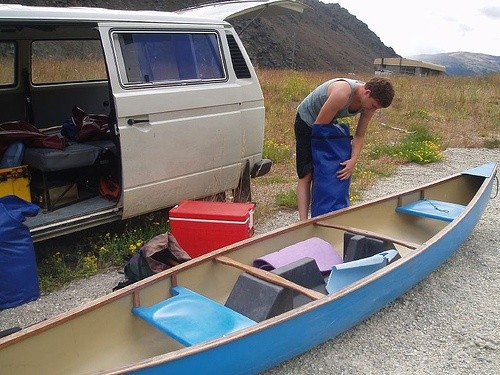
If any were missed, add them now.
[26,131,118,210]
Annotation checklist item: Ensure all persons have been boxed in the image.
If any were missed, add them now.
[294,76,394,221]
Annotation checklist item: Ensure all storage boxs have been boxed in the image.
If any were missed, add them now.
[167,199,256,259]
[0,164,32,204]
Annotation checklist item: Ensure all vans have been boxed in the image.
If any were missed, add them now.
[0,0,315,242]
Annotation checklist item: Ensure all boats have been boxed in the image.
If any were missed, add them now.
[0,161,498,375]
